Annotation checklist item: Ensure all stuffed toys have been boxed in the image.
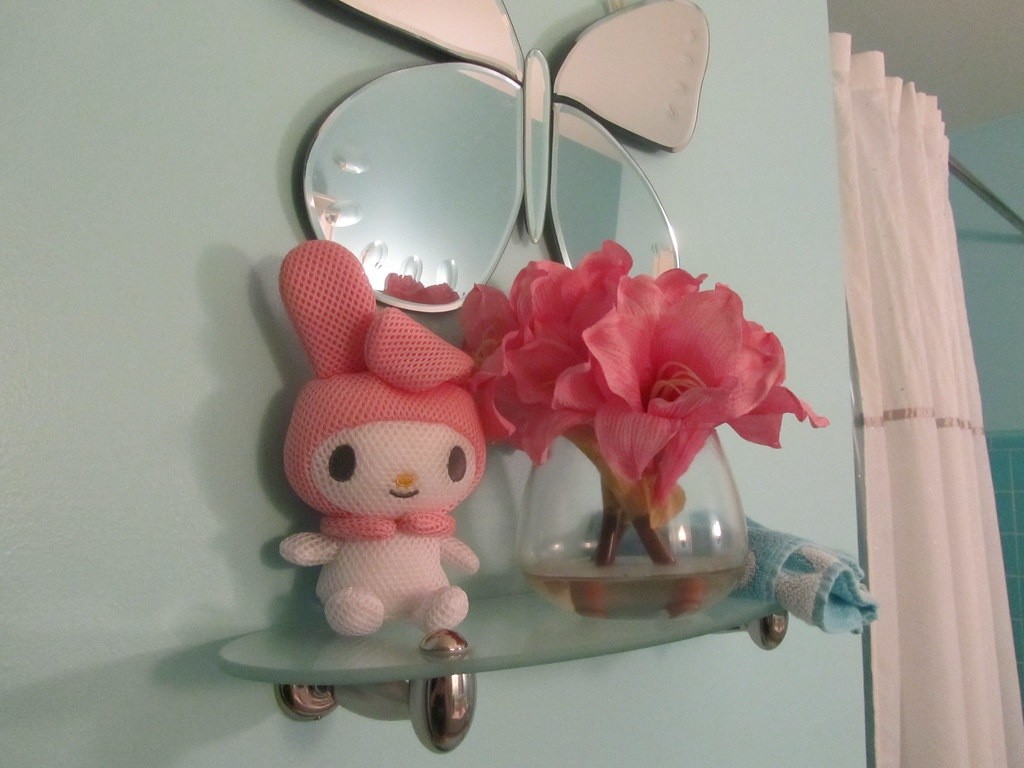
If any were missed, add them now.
[279,240,488,637]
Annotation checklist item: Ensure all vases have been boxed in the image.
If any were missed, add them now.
[515,422,748,620]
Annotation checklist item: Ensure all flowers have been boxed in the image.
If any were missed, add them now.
[378,272,460,305]
[462,240,831,566]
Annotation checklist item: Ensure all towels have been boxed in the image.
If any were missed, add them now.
[574,506,878,636]
